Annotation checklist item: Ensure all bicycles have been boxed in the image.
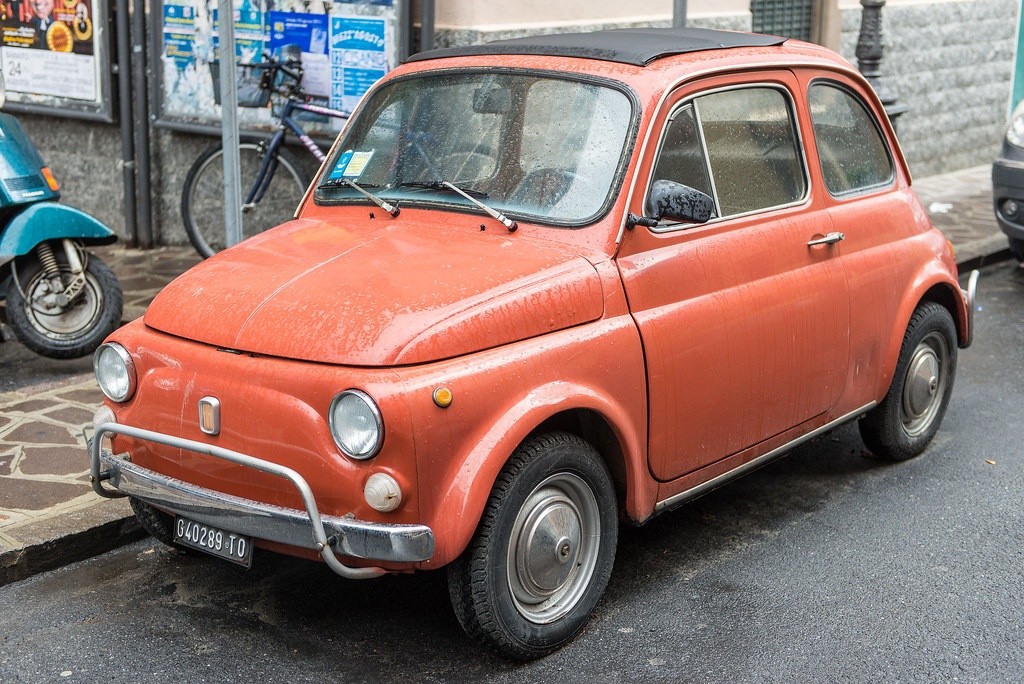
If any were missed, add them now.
[180,53,495,260]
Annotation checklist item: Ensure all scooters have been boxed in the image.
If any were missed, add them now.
[0,72,123,359]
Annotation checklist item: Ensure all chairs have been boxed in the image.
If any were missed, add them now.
[656,109,849,217]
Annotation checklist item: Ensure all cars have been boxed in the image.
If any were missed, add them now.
[86,28,981,660]
[991,99,1023,266]
